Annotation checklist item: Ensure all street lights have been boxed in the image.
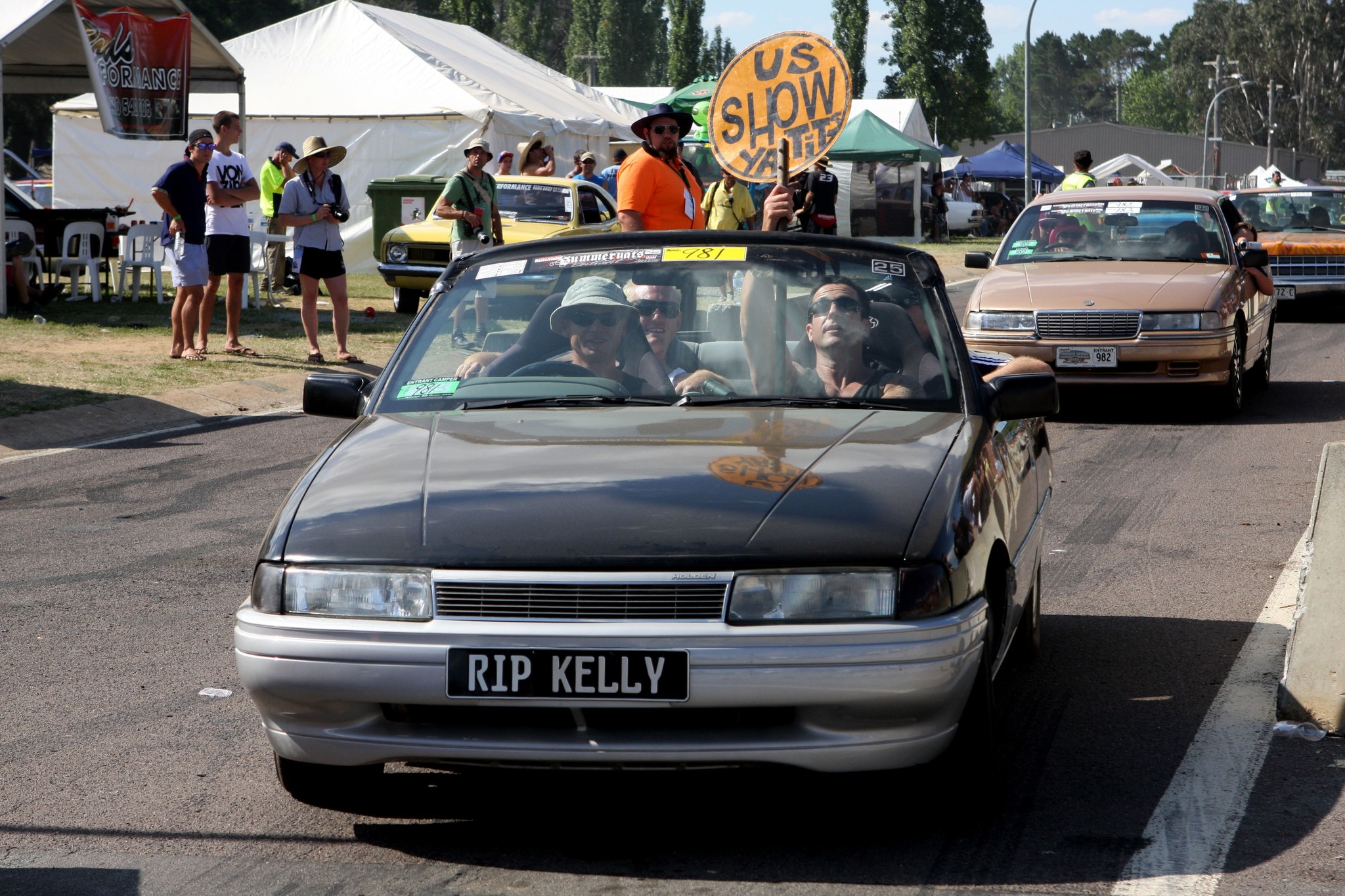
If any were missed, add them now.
[1202,55,1315,188]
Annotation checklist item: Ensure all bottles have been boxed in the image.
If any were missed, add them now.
[260,215,267,235]
[247,212,255,231]
[174,229,184,255]
[106,211,120,232]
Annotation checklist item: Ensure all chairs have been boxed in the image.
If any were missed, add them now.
[241,231,270,310]
[3,219,45,290]
[115,224,167,304]
[1285,214,1309,228]
[50,221,105,302]
[1309,205,1331,226]
[482,291,677,396]
[1163,225,1211,257]
[791,300,949,398]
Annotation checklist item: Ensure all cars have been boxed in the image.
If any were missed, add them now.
[2,172,119,288]
[379,175,620,316]
[960,184,1285,416]
[1216,185,1345,312]
[231,226,1062,832]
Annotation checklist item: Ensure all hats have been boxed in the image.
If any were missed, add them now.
[274,142,300,160]
[517,130,546,173]
[293,136,347,174]
[1038,211,1058,222]
[814,150,1137,184]
[497,148,628,163]
[1049,216,1088,253]
[185,129,213,158]
[463,138,493,163]
[630,103,693,141]
[549,276,640,337]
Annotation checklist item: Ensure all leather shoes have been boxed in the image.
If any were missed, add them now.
[473,331,488,350]
[451,333,475,348]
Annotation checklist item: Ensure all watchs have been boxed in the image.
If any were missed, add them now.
[496,240,504,245]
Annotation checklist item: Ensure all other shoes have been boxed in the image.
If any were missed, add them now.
[259,285,294,296]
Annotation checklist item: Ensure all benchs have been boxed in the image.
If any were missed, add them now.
[674,336,800,396]
[1115,232,1163,257]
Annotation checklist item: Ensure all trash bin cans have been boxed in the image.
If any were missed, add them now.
[366,174,452,263]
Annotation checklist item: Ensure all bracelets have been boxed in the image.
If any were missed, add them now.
[461,210,467,220]
[312,213,317,222]
[172,215,181,220]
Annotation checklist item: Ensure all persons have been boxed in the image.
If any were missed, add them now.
[194,111,261,358]
[435,139,505,351]
[616,103,706,231]
[738,185,927,404]
[150,128,217,362]
[278,136,366,366]
[452,274,736,396]
[525,275,664,398]
[43,0,1344,299]
[259,142,300,295]
[860,270,1056,385]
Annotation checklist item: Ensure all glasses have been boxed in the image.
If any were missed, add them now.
[629,299,680,319]
[807,297,869,323]
[649,124,680,135]
[1039,223,1081,239]
[582,159,594,164]
[192,143,216,150]
[314,151,331,158]
[566,311,630,326]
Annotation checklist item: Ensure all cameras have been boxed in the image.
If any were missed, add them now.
[328,202,348,222]
[462,218,490,246]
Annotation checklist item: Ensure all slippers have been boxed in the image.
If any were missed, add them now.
[308,353,325,363]
[170,345,258,362]
[339,353,364,363]
